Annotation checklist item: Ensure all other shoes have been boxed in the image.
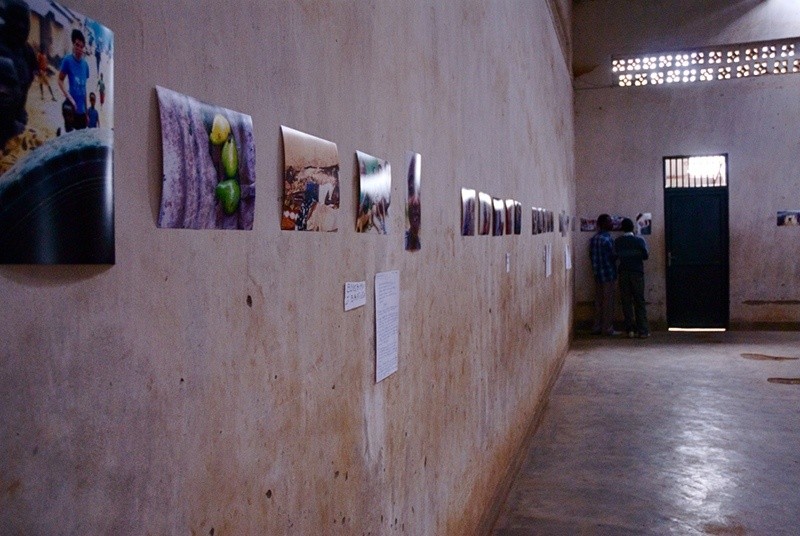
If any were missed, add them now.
[590,326,603,335]
[637,331,651,338]
[607,326,621,335]
[627,331,635,339]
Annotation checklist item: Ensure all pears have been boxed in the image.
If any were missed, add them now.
[211,113,240,213]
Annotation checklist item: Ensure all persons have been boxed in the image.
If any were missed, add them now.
[588,214,622,337]
[0,0,106,176]
[613,218,651,337]
[404,154,422,249]
